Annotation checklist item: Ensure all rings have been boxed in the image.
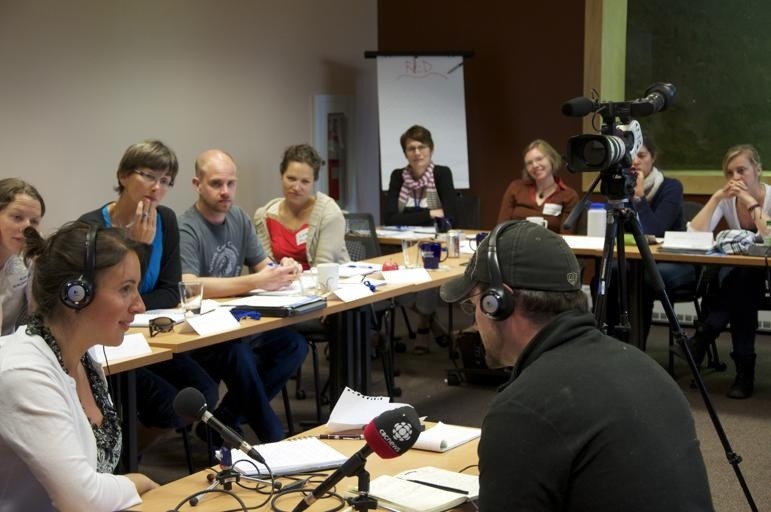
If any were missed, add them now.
[141,212,148,217]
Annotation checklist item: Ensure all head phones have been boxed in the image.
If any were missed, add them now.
[58,224,105,310]
[479,219,525,321]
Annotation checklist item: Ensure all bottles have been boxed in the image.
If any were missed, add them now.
[587,203,606,238]
[762,220,771,245]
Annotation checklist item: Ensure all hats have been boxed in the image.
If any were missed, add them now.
[438,222,580,303]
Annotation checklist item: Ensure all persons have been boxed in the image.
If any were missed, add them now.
[176,146,308,449]
[388,123,477,355]
[0,220,167,512]
[0,176,59,341]
[58,131,222,462]
[666,144,769,401]
[254,137,374,404]
[589,131,701,356]
[434,218,720,512]
[496,140,582,236]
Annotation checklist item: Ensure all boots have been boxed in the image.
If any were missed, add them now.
[672,321,720,366]
[729,351,756,399]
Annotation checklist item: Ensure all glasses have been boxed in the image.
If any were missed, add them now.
[459,292,488,317]
[131,169,172,188]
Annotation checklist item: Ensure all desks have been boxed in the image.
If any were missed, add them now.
[120,419,481,511]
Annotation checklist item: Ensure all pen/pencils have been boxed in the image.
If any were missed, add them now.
[126,212,147,229]
[414,231,435,234]
[315,435,365,440]
[348,265,373,268]
[407,480,469,494]
[268,262,281,267]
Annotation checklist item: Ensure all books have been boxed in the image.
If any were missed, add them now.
[347,465,480,512]
[214,437,349,480]
[411,421,482,453]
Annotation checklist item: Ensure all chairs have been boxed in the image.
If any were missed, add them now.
[447,194,479,228]
[295,213,401,399]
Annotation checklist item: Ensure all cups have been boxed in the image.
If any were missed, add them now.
[434,216,452,233]
[401,238,421,269]
[527,216,549,229]
[179,281,204,314]
[448,237,460,257]
[318,263,340,290]
[470,233,488,252]
[420,243,448,269]
[298,275,321,297]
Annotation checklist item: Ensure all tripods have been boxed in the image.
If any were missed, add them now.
[592,205,759,512]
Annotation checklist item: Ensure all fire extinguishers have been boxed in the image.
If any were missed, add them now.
[328,119,340,201]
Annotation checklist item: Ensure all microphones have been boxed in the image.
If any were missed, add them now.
[290,406,422,511]
[173,387,265,464]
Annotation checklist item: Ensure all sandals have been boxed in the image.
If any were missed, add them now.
[414,326,429,355]
[429,314,451,348]
